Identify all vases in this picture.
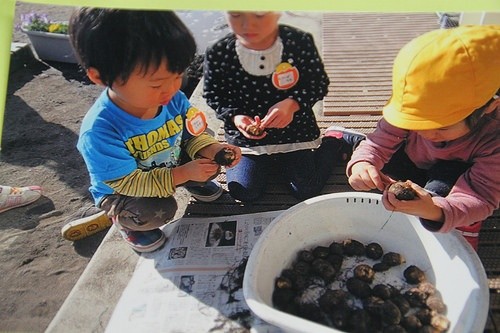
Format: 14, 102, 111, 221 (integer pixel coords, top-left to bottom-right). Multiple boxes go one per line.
21, 21, 85, 63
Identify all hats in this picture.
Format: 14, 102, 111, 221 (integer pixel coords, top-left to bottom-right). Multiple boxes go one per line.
382, 24, 500, 131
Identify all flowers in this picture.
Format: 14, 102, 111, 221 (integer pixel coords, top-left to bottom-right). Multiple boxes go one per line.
21, 14, 71, 35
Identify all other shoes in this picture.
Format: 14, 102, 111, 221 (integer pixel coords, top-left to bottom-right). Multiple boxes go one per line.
184, 179, 223, 203
0, 185, 42, 213
61, 205, 113, 241
456, 222, 480, 254
112, 216, 167, 253
322, 125, 368, 147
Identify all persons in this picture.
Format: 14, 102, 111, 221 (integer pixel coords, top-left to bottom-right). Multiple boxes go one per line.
201, 11, 368, 203
68, 7, 241, 253
0, 185, 42, 212
346, 23, 500, 252
